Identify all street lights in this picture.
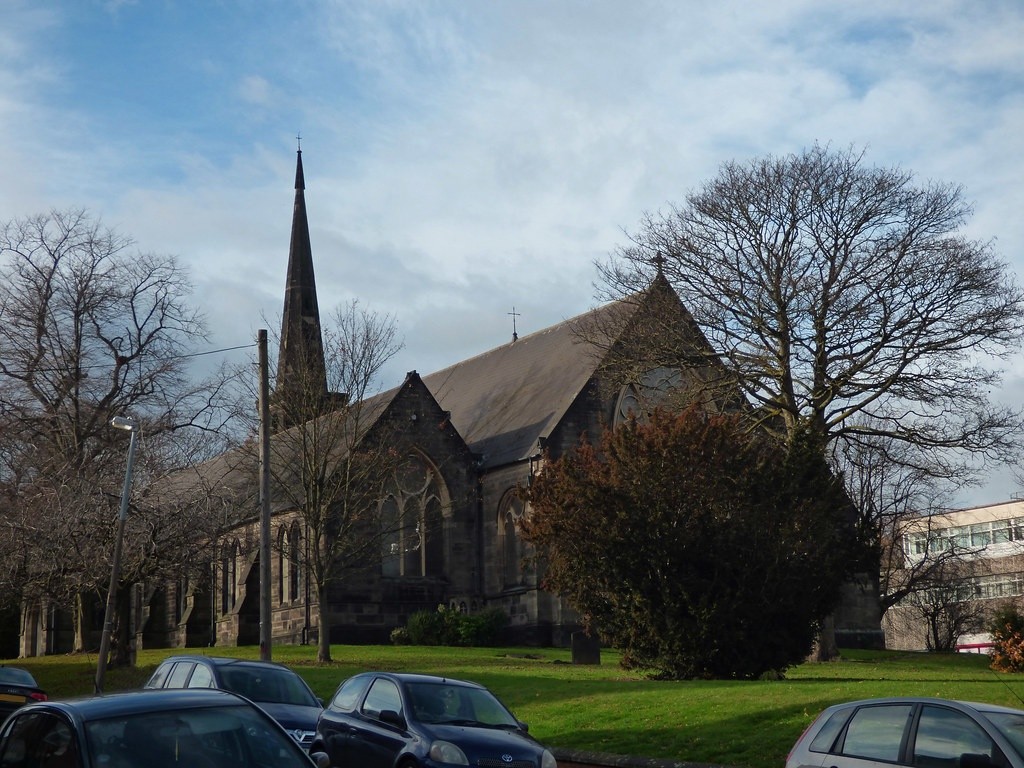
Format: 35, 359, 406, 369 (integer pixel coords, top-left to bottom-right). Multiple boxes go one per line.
90, 414, 140, 699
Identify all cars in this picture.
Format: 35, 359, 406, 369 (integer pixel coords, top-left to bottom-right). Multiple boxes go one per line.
0, 666, 48, 724
0, 685, 333, 768
307, 667, 558, 768
787, 693, 1024, 768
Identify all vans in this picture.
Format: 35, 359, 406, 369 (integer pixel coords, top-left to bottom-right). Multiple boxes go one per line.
143, 655, 328, 761
